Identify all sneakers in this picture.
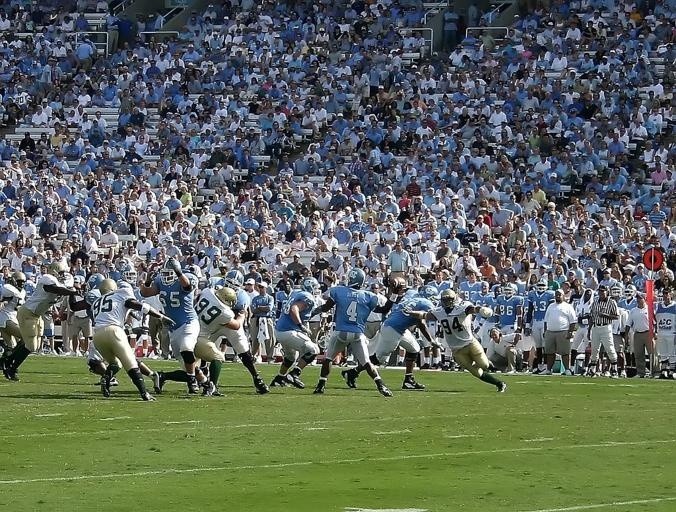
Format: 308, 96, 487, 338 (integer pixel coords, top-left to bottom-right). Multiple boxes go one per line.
35, 346, 414, 360
154, 371, 166, 394
186, 371, 228, 399
341, 369, 361, 388
421, 358, 676, 379
254, 366, 306, 395
373, 377, 395, 398
99, 375, 120, 398
141, 391, 158, 402
401, 373, 427, 391
313, 378, 326, 395
495, 378, 507, 393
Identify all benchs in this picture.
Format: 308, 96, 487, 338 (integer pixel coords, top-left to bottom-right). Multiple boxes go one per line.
1, 1, 676, 304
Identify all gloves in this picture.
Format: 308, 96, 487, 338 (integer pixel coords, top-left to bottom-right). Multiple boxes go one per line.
474, 303, 495, 319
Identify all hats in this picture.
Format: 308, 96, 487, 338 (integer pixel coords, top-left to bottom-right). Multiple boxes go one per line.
243, 275, 268, 288
1, 68, 616, 274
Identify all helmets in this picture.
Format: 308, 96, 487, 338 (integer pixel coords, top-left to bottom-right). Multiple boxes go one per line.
73, 272, 117, 294
300, 276, 322, 298
389, 276, 407, 297
215, 269, 245, 307
347, 267, 366, 288
502, 280, 638, 299
160, 258, 182, 286
420, 284, 440, 306
441, 288, 456, 310
